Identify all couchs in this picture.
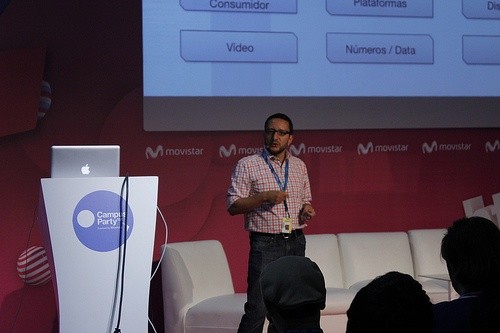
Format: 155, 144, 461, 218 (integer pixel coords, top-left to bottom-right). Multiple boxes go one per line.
303, 228, 460, 333
159, 240, 270, 333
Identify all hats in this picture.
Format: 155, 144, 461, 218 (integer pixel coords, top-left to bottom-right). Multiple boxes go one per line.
259, 255, 325, 309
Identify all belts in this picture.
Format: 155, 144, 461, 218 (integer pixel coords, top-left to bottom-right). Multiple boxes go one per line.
251, 229, 303, 239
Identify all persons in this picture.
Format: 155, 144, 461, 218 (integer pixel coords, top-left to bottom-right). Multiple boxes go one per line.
345, 216, 500, 333
259, 255, 326, 333
226, 113, 317, 333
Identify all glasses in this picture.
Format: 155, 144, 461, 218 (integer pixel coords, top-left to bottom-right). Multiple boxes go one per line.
265, 128, 291, 136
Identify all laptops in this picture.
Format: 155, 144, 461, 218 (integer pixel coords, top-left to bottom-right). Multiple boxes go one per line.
50, 146, 120, 178
